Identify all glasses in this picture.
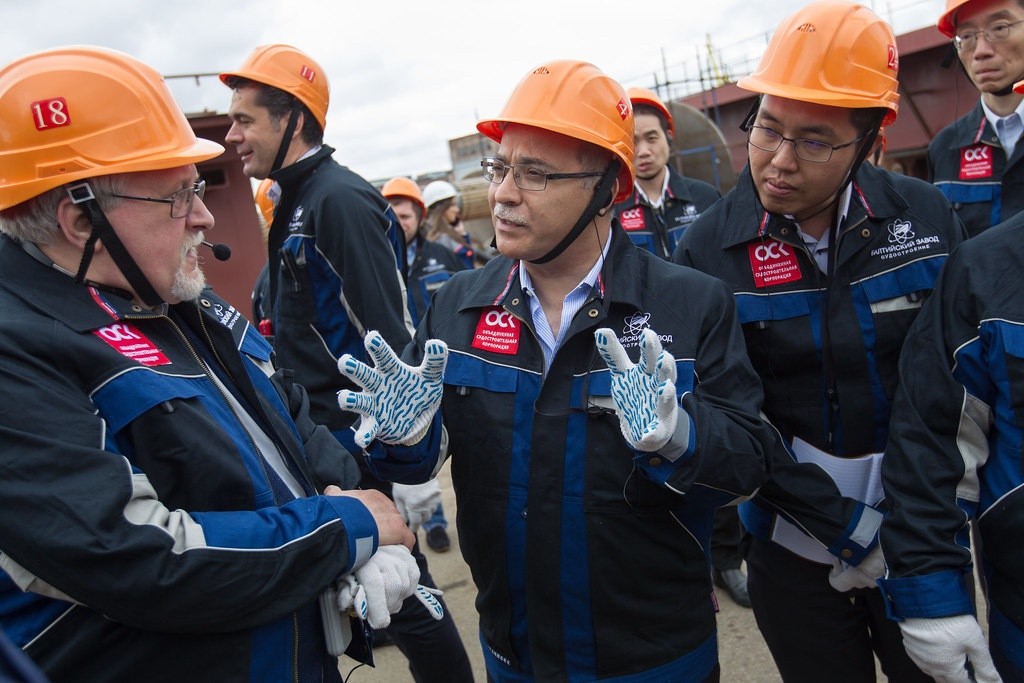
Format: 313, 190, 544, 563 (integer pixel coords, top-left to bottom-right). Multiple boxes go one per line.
744, 112, 866, 163
952, 20, 1024, 51
480, 157, 607, 191
104, 180, 206, 218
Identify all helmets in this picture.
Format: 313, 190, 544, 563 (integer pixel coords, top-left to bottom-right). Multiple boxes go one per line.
256, 179, 280, 225
627, 87, 674, 135
937, 0, 970, 38
219, 44, 328, 135
476, 59, 635, 204
381, 177, 425, 222
0, 44, 226, 208
422, 180, 456, 208
736, 1, 900, 126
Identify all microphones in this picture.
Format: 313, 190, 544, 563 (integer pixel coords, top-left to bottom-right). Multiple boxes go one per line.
200, 241, 231, 261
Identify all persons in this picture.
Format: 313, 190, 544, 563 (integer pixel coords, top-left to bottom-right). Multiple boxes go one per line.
880, 78, 1024, 683
380, 177, 472, 553
669, 0, 978, 683
338, 61, 775, 683
420, 181, 476, 268
0, 46, 416, 683
221, 45, 474, 683
254, 177, 279, 345
923, 0, 1024, 616
612, 87, 753, 609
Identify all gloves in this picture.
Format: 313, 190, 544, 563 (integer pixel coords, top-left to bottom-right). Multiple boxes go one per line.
394, 479, 442, 532
337, 330, 448, 447
596, 327, 678, 453
337, 542, 443, 628
898, 613, 1003, 683
828, 558, 879, 591
858, 541, 889, 583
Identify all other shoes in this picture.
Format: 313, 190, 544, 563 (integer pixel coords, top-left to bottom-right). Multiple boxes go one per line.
426, 527, 449, 551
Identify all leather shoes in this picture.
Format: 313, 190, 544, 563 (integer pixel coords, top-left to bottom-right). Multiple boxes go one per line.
713, 567, 752, 607
